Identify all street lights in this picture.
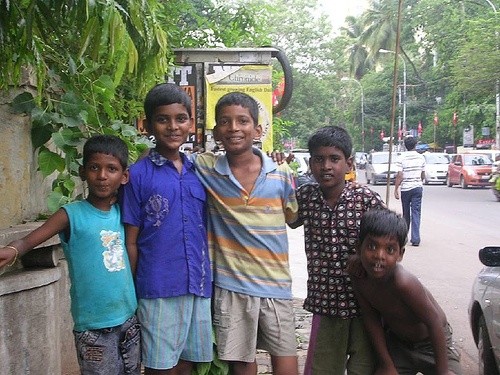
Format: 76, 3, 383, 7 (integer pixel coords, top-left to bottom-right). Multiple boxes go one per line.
378, 48, 407, 149
340, 77, 365, 154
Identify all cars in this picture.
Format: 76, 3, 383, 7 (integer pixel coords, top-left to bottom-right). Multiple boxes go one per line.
282, 148, 313, 175
341, 166, 358, 181
467, 246, 500, 375
352, 151, 402, 186
420, 152, 500, 202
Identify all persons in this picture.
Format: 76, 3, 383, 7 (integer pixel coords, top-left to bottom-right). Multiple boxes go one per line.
121, 83, 213, 375
268, 126, 388, 375
188, 92, 307, 375
288, 154, 301, 187
0, 134, 141, 375
350, 208, 465, 375
394, 138, 427, 246
